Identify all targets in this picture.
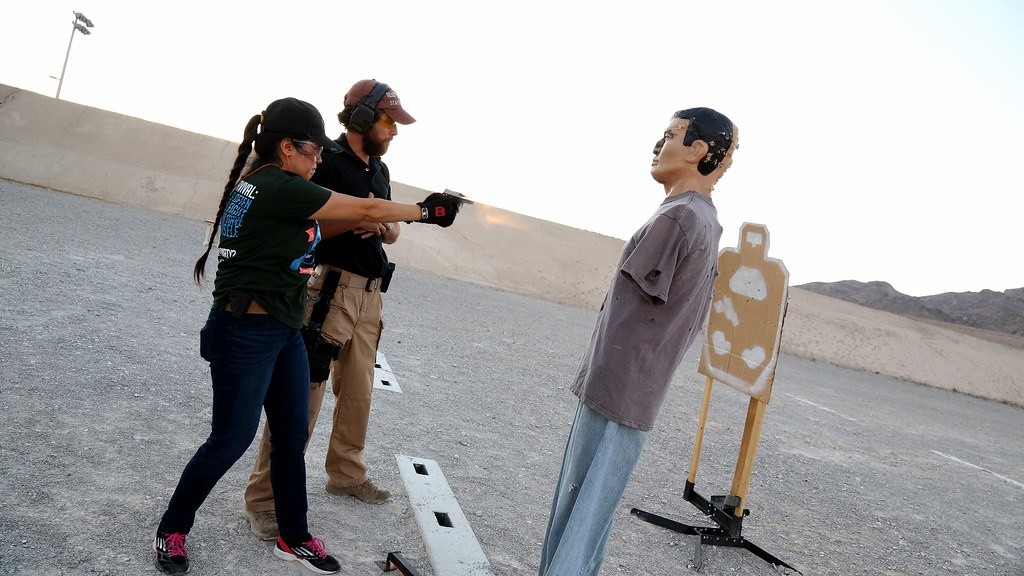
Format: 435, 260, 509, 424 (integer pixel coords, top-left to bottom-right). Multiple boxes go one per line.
630, 220, 806, 576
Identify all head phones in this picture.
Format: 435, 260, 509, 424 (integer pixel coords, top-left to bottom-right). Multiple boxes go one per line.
349, 83, 389, 133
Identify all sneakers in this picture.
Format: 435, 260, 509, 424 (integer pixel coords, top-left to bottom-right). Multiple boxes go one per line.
241, 503, 282, 542
152, 532, 192, 576
324, 477, 392, 505
272, 532, 342, 575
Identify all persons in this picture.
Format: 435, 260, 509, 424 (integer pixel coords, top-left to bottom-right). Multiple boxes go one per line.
243, 77, 417, 541
537, 107, 738, 576
153, 97, 474, 576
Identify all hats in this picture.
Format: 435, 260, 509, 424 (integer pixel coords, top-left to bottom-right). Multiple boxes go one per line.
258, 97, 346, 153
344, 81, 418, 128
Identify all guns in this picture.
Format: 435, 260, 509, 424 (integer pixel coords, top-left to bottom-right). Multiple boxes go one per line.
442, 188, 474, 217
